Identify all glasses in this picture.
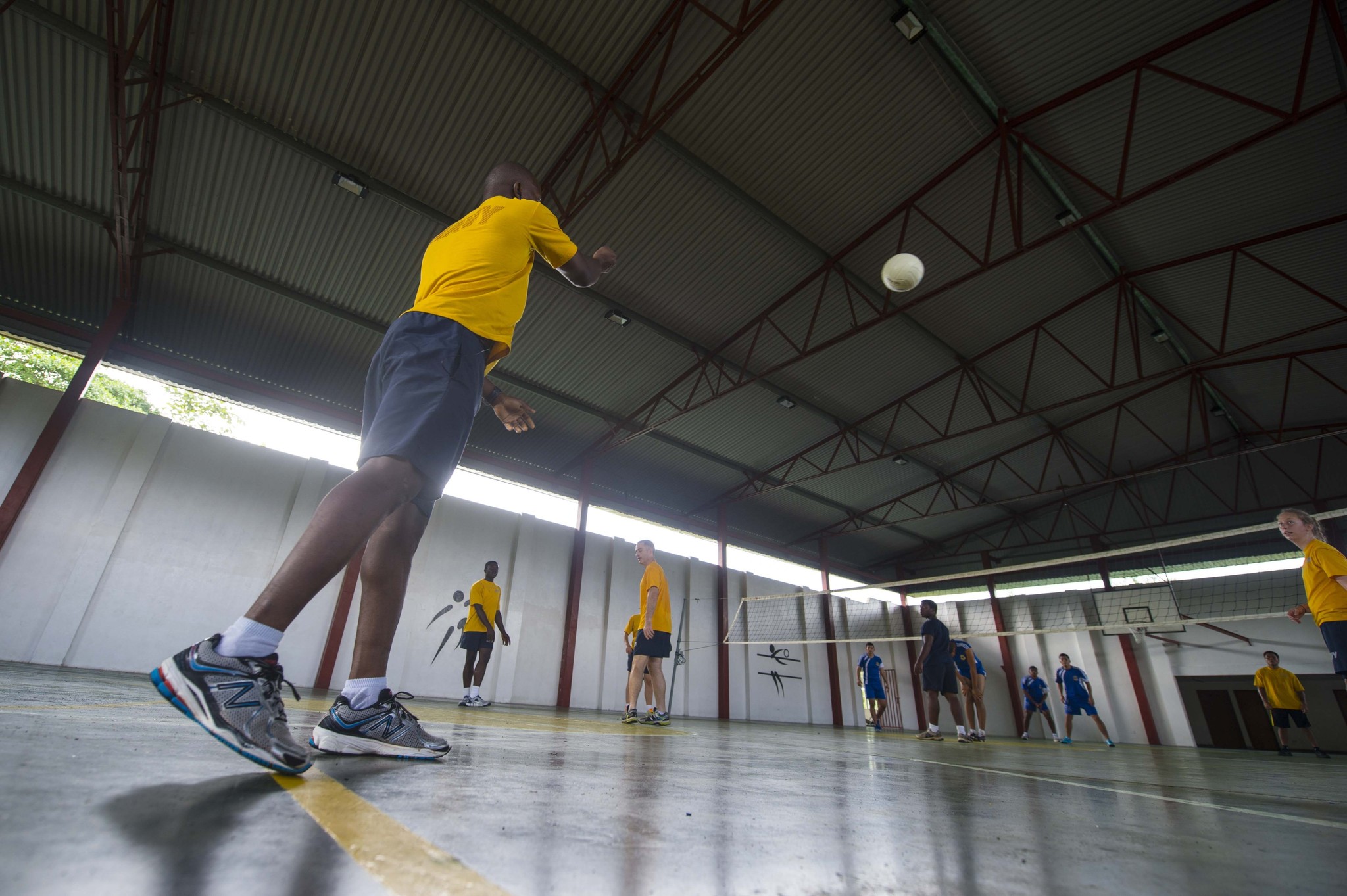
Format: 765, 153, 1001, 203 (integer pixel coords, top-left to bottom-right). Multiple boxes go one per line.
487, 567, 498, 570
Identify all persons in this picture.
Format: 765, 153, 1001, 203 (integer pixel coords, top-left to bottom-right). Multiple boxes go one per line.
913, 599, 974, 743
948, 639, 987, 742
620, 540, 672, 727
1276, 508, 1347, 686
1254, 651, 1332, 758
856, 642, 890, 731
1055, 653, 1115, 747
1021, 666, 1060, 742
150, 163, 614, 775
458, 561, 511, 707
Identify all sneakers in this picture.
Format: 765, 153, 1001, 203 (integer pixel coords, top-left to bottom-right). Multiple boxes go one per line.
638, 707, 670, 726
877, 719, 882, 730
458, 695, 470, 706
915, 728, 944, 741
957, 733, 974, 743
622, 704, 638, 724
875, 725, 881, 732
466, 695, 491, 707
148, 633, 316, 775
309, 688, 452, 759
645, 708, 654, 717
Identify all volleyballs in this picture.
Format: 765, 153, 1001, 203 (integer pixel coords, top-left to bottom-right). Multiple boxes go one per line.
882, 252, 925, 293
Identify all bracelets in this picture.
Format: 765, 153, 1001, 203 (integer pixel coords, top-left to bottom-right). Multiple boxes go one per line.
1298, 605, 1306, 613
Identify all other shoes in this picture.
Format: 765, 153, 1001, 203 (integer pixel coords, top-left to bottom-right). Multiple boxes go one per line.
1053, 737, 1060, 742
1022, 735, 1029, 740
1060, 736, 1073, 744
1106, 740, 1115, 747
978, 736, 986, 742
1278, 745, 1293, 756
968, 731, 979, 741
1313, 747, 1330, 758
